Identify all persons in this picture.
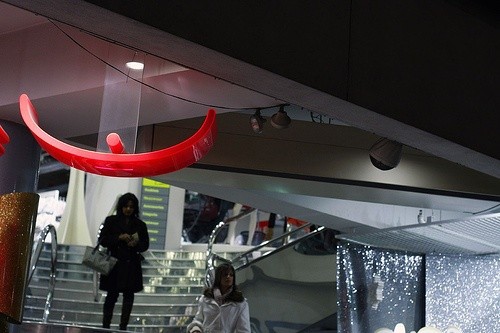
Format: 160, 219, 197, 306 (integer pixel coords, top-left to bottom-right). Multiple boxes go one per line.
96, 192, 149, 331
185, 263, 251, 333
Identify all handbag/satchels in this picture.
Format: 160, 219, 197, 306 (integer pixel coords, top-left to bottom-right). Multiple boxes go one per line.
81, 242, 119, 276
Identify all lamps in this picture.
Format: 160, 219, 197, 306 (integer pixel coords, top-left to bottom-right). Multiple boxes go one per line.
250, 109, 267, 136
270, 105, 290, 129
